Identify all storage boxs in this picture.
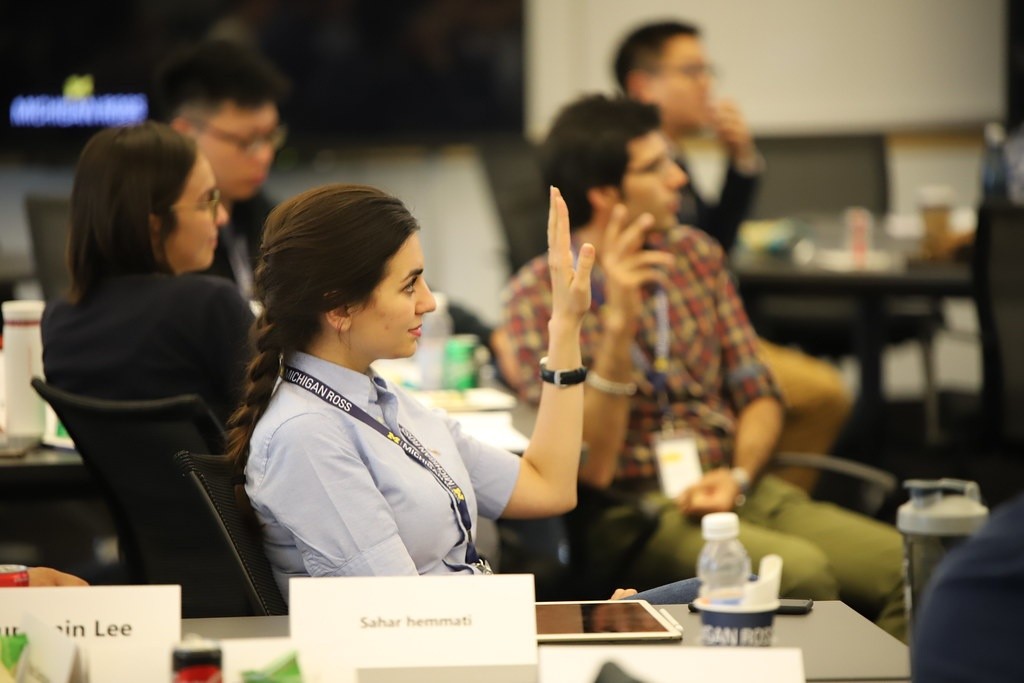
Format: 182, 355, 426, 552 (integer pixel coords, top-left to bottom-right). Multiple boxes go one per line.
286, 573, 539, 683
0, 583, 179, 683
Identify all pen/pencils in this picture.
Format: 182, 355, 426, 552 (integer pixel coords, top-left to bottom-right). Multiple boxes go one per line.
659, 608, 684, 632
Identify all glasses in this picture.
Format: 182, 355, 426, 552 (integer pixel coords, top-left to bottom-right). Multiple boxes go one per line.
655, 63, 717, 78
168, 189, 220, 224
629, 147, 675, 178
183, 116, 290, 154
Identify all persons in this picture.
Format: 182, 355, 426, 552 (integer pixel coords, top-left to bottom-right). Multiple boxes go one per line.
607, 13, 852, 496
39, 121, 284, 427
493, 93, 932, 643
905, 494, 1024, 683
134, 60, 290, 322
223, 184, 759, 616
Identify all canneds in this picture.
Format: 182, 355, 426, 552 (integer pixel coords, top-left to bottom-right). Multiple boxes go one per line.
0, 564, 30, 588
443, 334, 478, 390
169, 633, 223, 683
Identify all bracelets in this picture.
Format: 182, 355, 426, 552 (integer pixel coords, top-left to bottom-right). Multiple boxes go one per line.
589, 368, 640, 398
539, 358, 589, 389
729, 464, 752, 492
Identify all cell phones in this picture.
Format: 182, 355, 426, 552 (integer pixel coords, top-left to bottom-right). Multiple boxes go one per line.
688, 597, 814, 615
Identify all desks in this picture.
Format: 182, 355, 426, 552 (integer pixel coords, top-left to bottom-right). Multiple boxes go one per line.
723, 229, 978, 478
180, 596, 910, 683
0, 369, 587, 495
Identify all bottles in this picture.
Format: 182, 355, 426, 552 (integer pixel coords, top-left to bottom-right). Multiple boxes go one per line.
697, 511, 752, 609
0, 300, 46, 439
896, 477, 990, 638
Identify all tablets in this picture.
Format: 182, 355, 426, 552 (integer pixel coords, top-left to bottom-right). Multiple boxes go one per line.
535, 599, 682, 643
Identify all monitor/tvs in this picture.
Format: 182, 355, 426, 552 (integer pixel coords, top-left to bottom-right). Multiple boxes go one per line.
0, 0, 527, 151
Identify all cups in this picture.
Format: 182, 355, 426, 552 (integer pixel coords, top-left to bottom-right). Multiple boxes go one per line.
917, 183, 955, 258
691, 597, 782, 647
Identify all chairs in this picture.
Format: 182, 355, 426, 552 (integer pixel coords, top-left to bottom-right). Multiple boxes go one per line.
175, 441, 287, 618
28, 379, 244, 618
